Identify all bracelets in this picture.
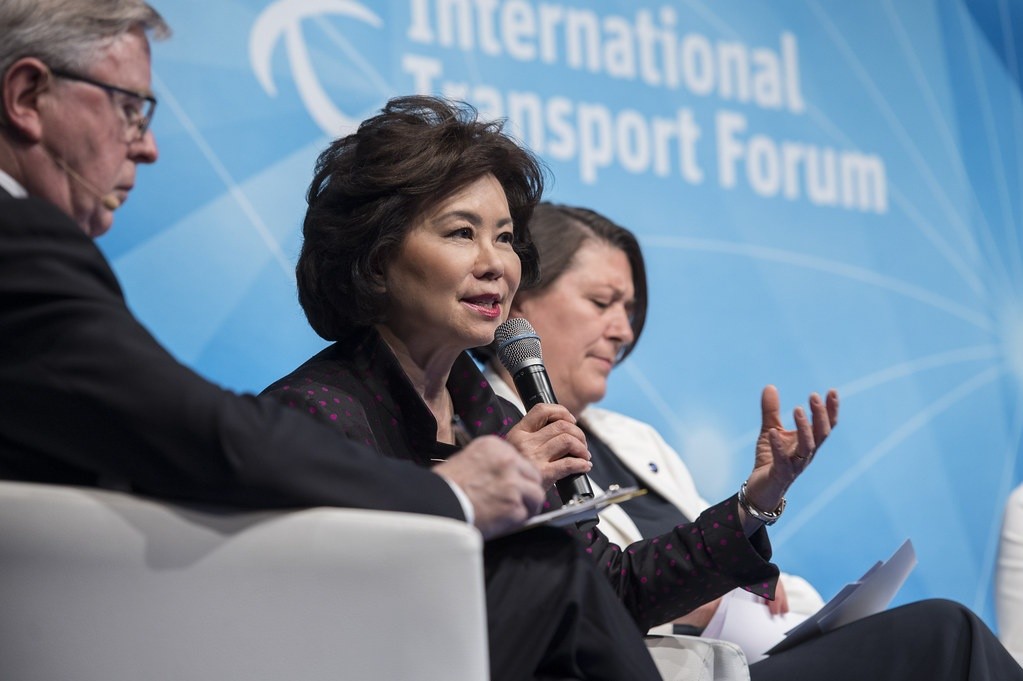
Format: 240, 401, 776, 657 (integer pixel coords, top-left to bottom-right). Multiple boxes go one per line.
740, 481, 787, 526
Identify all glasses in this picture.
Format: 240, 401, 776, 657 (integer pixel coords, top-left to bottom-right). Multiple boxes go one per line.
52, 67, 157, 139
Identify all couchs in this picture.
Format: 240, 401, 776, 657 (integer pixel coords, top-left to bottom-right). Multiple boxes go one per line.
0, 481, 825, 681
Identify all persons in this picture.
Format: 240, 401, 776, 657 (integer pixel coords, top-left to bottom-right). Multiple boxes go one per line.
0, 0, 662, 681
259, 96, 840, 637
470, 202, 1023, 681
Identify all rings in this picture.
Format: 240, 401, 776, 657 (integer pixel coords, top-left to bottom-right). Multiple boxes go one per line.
793, 453, 812, 464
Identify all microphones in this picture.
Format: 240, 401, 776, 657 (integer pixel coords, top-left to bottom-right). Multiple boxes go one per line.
495, 317, 600, 533
38, 142, 122, 213
